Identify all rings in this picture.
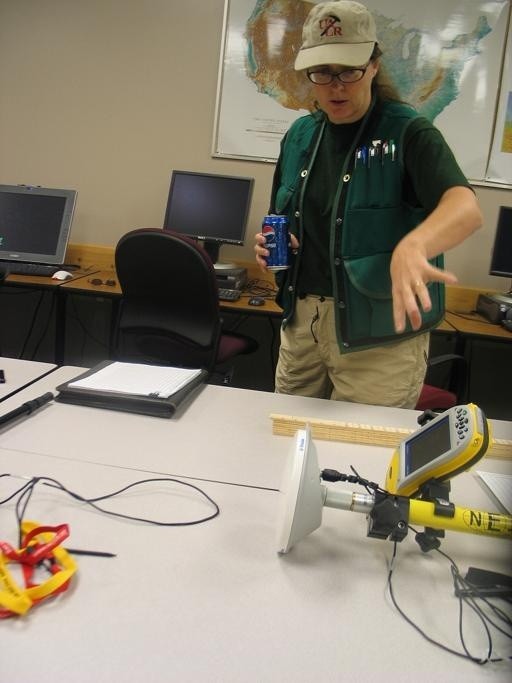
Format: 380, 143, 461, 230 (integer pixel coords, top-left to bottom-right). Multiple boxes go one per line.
413, 280, 424, 289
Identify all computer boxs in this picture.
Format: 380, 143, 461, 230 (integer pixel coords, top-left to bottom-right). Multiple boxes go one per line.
215, 269, 248, 291
476, 294, 512, 326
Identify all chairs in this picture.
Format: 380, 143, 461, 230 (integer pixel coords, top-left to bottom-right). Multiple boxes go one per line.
413, 382, 459, 412
114, 226, 260, 381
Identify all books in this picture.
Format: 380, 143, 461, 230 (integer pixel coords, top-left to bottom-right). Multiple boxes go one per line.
53, 359, 208, 419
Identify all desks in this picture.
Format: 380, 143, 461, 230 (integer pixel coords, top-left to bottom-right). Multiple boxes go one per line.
0, 244, 110, 294
444, 282, 512, 404
59, 242, 459, 402
0, 356, 512, 683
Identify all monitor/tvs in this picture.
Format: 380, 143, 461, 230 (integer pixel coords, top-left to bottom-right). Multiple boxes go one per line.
162, 170, 256, 269
476, 205, 512, 303
0, 186, 79, 265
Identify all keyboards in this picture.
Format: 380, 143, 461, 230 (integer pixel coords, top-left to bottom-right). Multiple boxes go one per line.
218, 288, 242, 302
0, 259, 59, 275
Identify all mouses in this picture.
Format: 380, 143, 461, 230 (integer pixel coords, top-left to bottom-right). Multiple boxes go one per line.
51, 270, 74, 280
249, 297, 265, 306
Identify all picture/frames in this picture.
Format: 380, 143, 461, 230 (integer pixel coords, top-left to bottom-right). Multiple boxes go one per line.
210, 0, 512, 190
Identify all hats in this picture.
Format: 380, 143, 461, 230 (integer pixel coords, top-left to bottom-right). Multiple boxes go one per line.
293, 1, 379, 73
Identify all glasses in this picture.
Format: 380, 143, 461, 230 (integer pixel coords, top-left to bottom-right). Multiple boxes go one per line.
305, 53, 377, 86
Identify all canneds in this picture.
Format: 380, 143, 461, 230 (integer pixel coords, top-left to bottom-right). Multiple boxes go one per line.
262, 216, 291, 272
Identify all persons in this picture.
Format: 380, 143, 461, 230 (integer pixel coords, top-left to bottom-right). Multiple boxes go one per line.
254, 1, 485, 410
